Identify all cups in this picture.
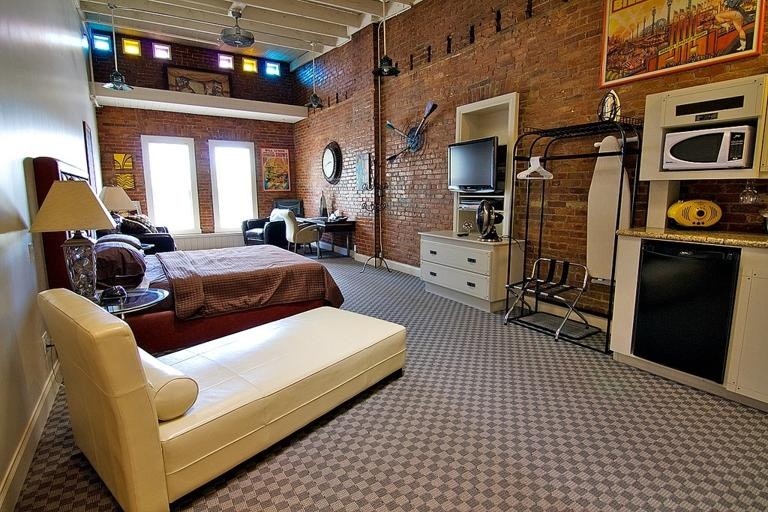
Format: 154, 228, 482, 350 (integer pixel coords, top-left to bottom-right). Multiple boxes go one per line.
336, 210, 343, 216
464, 217, 473, 234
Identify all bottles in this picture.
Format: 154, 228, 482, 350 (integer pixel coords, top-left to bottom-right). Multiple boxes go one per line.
329, 191, 336, 212
318, 190, 327, 216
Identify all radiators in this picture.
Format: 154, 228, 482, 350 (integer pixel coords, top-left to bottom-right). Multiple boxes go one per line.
173, 232, 244, 250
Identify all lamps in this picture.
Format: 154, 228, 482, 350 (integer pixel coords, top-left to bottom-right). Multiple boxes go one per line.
27, 180, 117, 304
102, 4, 134, 92
372, 0, 400, 76
99, 187, 137, 232
221, 11, 254, 47
304, 42, 325, 109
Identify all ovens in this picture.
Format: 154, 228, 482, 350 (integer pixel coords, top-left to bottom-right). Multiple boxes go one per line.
633, 239, 742, 386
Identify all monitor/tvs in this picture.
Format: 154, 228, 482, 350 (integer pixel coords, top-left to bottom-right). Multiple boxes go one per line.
447, 136, 499, 194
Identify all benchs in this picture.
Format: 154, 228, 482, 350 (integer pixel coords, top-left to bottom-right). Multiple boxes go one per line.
37, 288, 407, 512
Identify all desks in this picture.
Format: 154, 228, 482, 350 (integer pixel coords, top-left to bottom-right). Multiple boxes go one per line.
303, 218, 356, 258
94, 289, 169, 318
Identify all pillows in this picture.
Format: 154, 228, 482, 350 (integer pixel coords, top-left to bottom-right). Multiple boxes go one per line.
96, 234, 145, 257
97, 242, 147, 288
125, 215, 159, 233
137, 346, 199, 422
269, 208, 288, 222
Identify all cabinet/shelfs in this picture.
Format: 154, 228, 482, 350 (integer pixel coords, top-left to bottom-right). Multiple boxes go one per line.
417, 231, 525, 314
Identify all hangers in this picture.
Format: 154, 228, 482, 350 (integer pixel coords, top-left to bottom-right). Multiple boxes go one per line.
516, 157, 553, 180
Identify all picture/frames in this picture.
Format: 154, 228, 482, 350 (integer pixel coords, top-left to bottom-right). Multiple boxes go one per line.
599, 0, 766, 89
83, 121, 97, 191
163, 64, 233, 98
261, 148, 292, 192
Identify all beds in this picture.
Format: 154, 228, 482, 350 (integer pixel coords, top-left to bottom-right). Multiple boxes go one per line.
33, 157, 345, 354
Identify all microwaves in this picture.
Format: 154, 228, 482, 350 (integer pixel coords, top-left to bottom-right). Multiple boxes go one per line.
662, 125, 753, 171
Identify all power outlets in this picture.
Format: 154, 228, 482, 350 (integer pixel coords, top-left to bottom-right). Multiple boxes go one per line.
41, 331, 52, 359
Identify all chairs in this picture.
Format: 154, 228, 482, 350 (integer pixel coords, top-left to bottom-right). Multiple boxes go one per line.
241, 209, 287, 249
122, 215, 176, 254
503, 258, 601, 341
280, 211, 322, 260
